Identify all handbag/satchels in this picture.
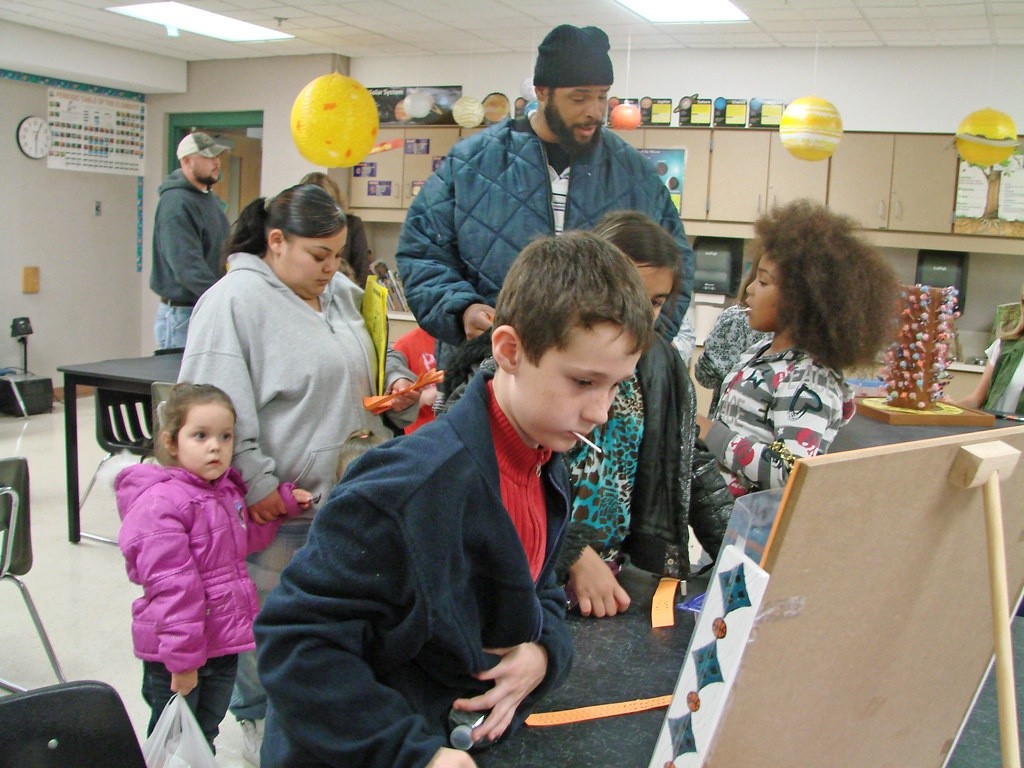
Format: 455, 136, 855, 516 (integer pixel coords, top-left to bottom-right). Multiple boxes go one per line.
145, 695, 219, 768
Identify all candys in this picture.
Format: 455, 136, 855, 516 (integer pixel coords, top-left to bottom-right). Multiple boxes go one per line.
878, 285, 961, 408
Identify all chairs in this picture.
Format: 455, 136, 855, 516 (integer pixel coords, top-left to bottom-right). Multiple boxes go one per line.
0, 457, 68, 694
79, 388, 156, 546
0, 680, 146, 768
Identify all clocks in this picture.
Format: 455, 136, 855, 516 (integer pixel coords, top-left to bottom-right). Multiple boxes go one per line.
17, 116, 53, 159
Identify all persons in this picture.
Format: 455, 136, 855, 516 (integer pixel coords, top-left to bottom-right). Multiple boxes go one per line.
148, 132, 234, 356
940, 279, 1024, 415
445, 200, 750, 618
250, 229, 655, 768
696, 194, 903, 571
396, 24, 696, 418
176, 182, 430, 768
385, 324, 439, 436
112, 379, 315, 757
297, 172, 373, 291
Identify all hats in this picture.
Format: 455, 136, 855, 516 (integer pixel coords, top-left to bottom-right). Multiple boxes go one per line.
176, 132, 231, 158
533, 24, 614, 86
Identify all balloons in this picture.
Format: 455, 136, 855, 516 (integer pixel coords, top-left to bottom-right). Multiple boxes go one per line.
403, 92, 433, 117
609, 103, 643, 130
779, 96, 843, 162
955, 109, 1017, 167
290, 71, 380, 169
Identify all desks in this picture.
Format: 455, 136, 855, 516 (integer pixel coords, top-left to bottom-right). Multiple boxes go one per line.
56, 353, 185, 545
466, 565, 1024, 768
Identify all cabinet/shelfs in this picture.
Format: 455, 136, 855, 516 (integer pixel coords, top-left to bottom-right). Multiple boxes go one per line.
347, 122, 1024, 254
